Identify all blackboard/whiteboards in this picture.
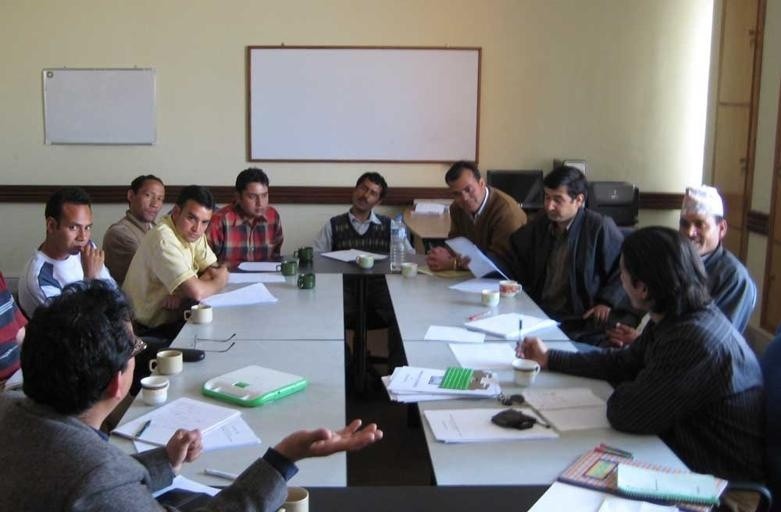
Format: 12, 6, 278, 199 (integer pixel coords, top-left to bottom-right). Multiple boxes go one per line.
42, 67, 156, 145
247, 47, 481, 164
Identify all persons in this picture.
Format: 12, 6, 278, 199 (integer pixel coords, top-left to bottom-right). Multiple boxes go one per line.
598, 181, 758, 352
422, 159, 529, 273
119, 184, 229, 345
204, 166, 285, 268
512, 222, 768, 482
504, 164, 629, 332
16, 187, 166, 380
301, 169, 413, 256
101, 172, 165, 283
0, 269, 27, 392
0, 275, 385, 512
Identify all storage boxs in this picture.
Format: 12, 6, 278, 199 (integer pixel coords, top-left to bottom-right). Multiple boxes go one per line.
400, 206, 453, 255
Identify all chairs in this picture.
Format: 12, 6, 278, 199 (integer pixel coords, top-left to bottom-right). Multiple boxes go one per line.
718, 328, 781, 512
735, 278, 757, 336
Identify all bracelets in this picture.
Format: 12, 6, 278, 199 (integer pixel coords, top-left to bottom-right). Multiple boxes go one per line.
450, 254, 466, 270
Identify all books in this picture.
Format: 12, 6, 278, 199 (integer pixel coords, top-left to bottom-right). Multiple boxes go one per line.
613, 464, 720, 507
465, 311, 560, 340
556, 450, 729, 511
111, 396, 242, 447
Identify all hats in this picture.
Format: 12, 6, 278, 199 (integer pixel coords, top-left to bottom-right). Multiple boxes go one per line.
679, 184, 724, 217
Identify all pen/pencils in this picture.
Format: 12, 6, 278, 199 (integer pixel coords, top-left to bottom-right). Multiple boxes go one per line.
136, 419, 151, 437
519, 316, 523, 347
428, 241, 434, 250
601, 442, 633, 455
538, 421, 549, 428
203, 468, 237, 481
594, 446, 632, 458
469, 310, 491, 321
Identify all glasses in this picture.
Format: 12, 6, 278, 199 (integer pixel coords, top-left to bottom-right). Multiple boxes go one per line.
101, 333, 147, 392
193, 334, 236, 353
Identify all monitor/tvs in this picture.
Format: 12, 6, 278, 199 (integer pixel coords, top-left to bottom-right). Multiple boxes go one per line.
486, 168, 544, 212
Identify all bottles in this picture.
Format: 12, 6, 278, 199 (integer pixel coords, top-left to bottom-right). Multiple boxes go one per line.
389, 215, 405, 272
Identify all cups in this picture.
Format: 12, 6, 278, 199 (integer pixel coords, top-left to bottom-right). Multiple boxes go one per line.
276, 259, 298, 276
499, 280, 522, 297
149, 350, 183, 375
294, 247, 314, 263
140, 376, 169, 405
400, 262, 417, 278
183, 304, 213, 324
511, 359, 541, 386
356, 255, 375, 269
276, 486, 309, 512
298, 273, 316, 289
481, 289, 500, 307
